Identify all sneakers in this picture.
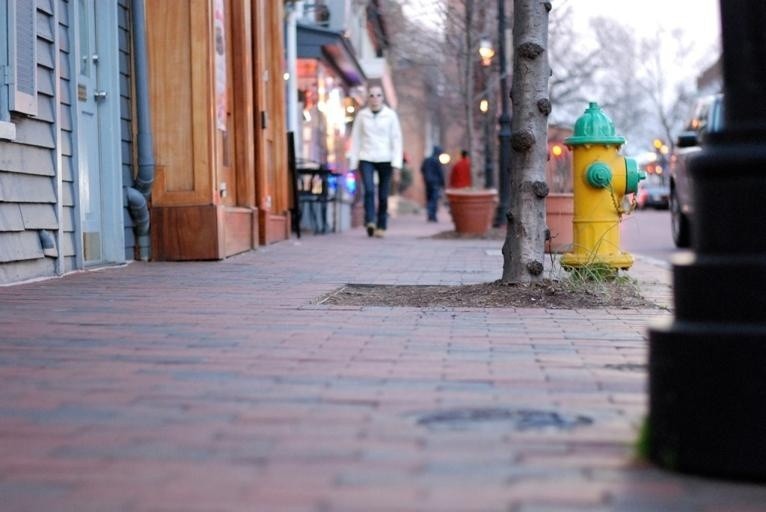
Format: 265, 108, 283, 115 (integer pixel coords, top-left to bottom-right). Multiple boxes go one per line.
366, 223, 384, 237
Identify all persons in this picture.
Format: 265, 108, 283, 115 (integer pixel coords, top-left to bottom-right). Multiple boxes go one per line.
421, 142, 447, 222
449, 148, 473, 189
349, 83, 404, 239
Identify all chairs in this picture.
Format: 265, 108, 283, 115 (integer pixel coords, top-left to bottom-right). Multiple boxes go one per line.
295, 168, 343, 233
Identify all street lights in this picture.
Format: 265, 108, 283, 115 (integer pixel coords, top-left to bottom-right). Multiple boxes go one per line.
479, 47, 495, 189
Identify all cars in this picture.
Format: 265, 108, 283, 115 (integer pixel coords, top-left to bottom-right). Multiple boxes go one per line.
638, 92, 724, 250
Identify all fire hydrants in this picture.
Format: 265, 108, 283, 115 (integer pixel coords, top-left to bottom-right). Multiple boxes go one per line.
559, 101, 646, 284
